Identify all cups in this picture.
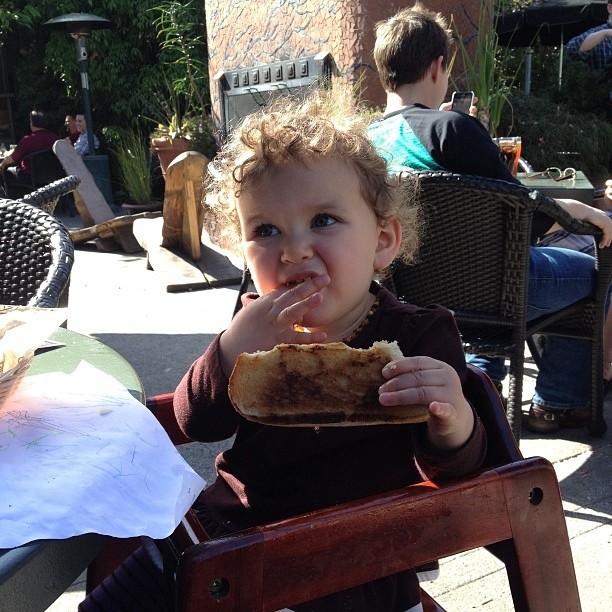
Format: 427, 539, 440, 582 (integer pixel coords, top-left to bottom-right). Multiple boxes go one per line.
491, 136, 517, 175
500, 136, 522, 173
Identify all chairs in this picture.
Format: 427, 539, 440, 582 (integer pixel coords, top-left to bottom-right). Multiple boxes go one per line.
0, 199, 74, 308
181, 456, 578, 610
20, 173, 81, 214
86, 391, 213, 599
381, 170, 611, 450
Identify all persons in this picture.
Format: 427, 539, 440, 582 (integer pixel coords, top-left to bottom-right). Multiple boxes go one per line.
77, 89, 503, 612
0, 111, 59, 191
64, 113, 81, 147
534, 178, 612, 399
566, 0, 612, 71
363, 2, 612, 433
74, 113, 100, 157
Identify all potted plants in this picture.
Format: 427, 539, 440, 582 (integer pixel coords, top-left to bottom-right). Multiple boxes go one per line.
105, 126, 160, 216
138, 71, 203, 184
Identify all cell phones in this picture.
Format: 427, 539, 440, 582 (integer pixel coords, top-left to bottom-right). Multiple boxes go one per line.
451, 90, 474, 117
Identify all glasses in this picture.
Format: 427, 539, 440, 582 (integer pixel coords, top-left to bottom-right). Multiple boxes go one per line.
526, 166, 577, 181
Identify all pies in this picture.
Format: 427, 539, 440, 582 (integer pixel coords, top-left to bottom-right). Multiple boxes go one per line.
226, 339, 433, 428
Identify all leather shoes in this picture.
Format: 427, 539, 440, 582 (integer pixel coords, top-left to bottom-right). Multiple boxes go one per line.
492, 380, 507, 413
526, 404, 594, 434
